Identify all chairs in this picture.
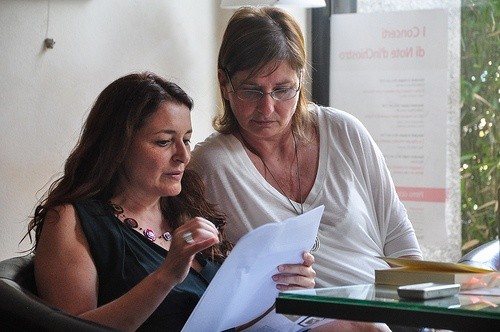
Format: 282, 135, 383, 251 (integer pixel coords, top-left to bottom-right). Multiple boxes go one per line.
0, 254, 123, 332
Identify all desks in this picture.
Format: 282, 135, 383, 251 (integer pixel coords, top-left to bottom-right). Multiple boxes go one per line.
275, 283, 500, 332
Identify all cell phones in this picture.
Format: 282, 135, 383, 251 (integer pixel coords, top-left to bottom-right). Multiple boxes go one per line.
396, 283, 460, 300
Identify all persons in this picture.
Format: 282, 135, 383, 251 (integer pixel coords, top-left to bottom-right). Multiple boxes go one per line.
19, 72, 393, 332
374, 256, 500, 290
184, 6, 455, 332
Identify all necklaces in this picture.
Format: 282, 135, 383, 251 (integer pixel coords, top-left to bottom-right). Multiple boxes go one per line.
112, 203, 172, 243
243, 128, 321, 253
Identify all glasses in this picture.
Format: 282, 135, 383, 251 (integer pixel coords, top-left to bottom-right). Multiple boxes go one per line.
223, 69, 301, 102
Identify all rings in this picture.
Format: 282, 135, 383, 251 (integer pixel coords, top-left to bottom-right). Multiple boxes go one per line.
182, 233, 194, 244
